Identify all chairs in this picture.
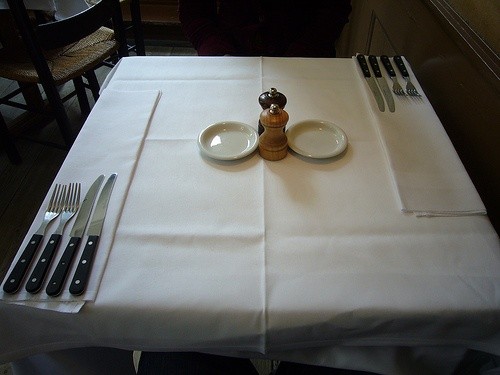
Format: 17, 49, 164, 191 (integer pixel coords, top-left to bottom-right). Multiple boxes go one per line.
1, 0, 130, 164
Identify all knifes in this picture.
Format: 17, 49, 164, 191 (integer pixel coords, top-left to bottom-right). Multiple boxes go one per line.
357, 54, 385, 112
368, 55, 395, 112
46, 175, 106, 297
69, 173, 118, 297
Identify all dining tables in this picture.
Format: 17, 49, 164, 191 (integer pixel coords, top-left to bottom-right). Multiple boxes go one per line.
0, 54, 500, 361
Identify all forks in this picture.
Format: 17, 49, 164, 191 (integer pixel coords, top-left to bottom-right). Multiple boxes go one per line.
3, 183, 67, 293
25, 182, 81, 294
380, 55, 408, 96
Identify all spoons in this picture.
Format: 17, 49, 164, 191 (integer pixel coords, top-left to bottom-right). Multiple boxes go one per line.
393, 56, 422, 96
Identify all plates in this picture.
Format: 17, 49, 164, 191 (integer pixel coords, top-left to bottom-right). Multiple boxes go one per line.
285, 119, 347, 159
199, 121, 260, 161
